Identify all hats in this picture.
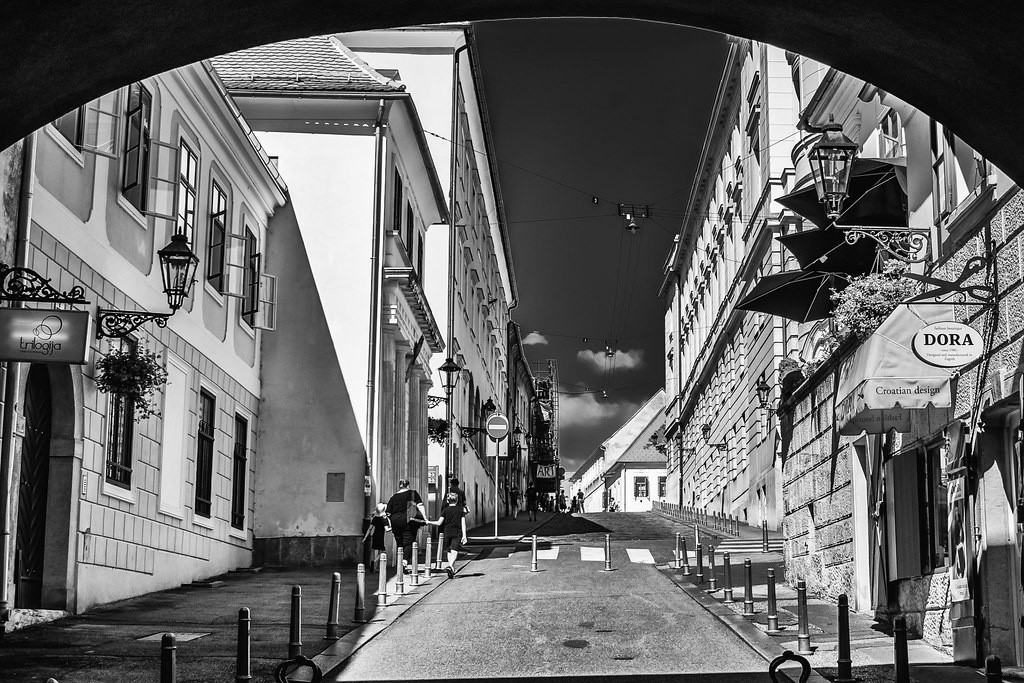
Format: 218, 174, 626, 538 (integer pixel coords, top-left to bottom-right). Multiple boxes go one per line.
373, 503, 387, 517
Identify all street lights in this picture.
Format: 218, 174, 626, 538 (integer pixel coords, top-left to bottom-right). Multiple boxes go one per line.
437, 358, 462, 497
524, 433, 534, 487
513, 427, 522, 487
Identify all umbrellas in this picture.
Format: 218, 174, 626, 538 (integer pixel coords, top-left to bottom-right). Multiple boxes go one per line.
736, 157, 908, 322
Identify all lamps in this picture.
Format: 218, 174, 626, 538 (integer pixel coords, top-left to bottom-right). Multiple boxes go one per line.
428, 355, 462, 409
651, 432, 669, 457
756, 381, 775, 411
96, 226, 199, 340
807, 112, 933, 268
522, 432, 534, 450
675, 433, 696, 455
701, 424, 728, 453
509, 426, 523, 454
461, 395, 496, 437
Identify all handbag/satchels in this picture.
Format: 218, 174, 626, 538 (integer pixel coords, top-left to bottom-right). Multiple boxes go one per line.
406, 490, 426, 526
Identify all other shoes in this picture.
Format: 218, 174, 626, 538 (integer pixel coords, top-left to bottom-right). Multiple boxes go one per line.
370, 560, 377, 574
446, 566, 453, 579
402, 562, 409, 574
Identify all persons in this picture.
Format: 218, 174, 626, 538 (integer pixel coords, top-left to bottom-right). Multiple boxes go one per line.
609, 497, 616, 512
362, 479, 470, 579
511, 482, 585, 522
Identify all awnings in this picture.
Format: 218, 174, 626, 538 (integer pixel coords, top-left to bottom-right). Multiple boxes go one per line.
834, 286, 956, 434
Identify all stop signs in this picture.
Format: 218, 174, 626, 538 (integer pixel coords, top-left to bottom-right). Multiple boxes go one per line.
488, 415, 509, 440
